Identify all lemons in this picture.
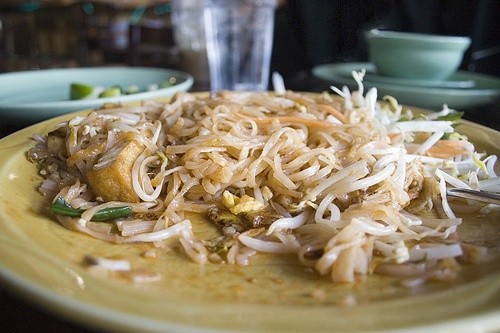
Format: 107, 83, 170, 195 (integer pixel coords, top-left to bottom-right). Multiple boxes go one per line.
70, 83, 121, 99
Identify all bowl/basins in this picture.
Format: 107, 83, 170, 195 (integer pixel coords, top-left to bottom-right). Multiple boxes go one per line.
363, 29, 471, 81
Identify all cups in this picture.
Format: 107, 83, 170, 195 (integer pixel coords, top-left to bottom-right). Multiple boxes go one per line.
171, 0, 272, 90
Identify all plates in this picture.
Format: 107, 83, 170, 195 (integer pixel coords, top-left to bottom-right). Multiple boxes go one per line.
0, 67, 196, 119
0, 91, 500, 333
311, 62, 500, 108
